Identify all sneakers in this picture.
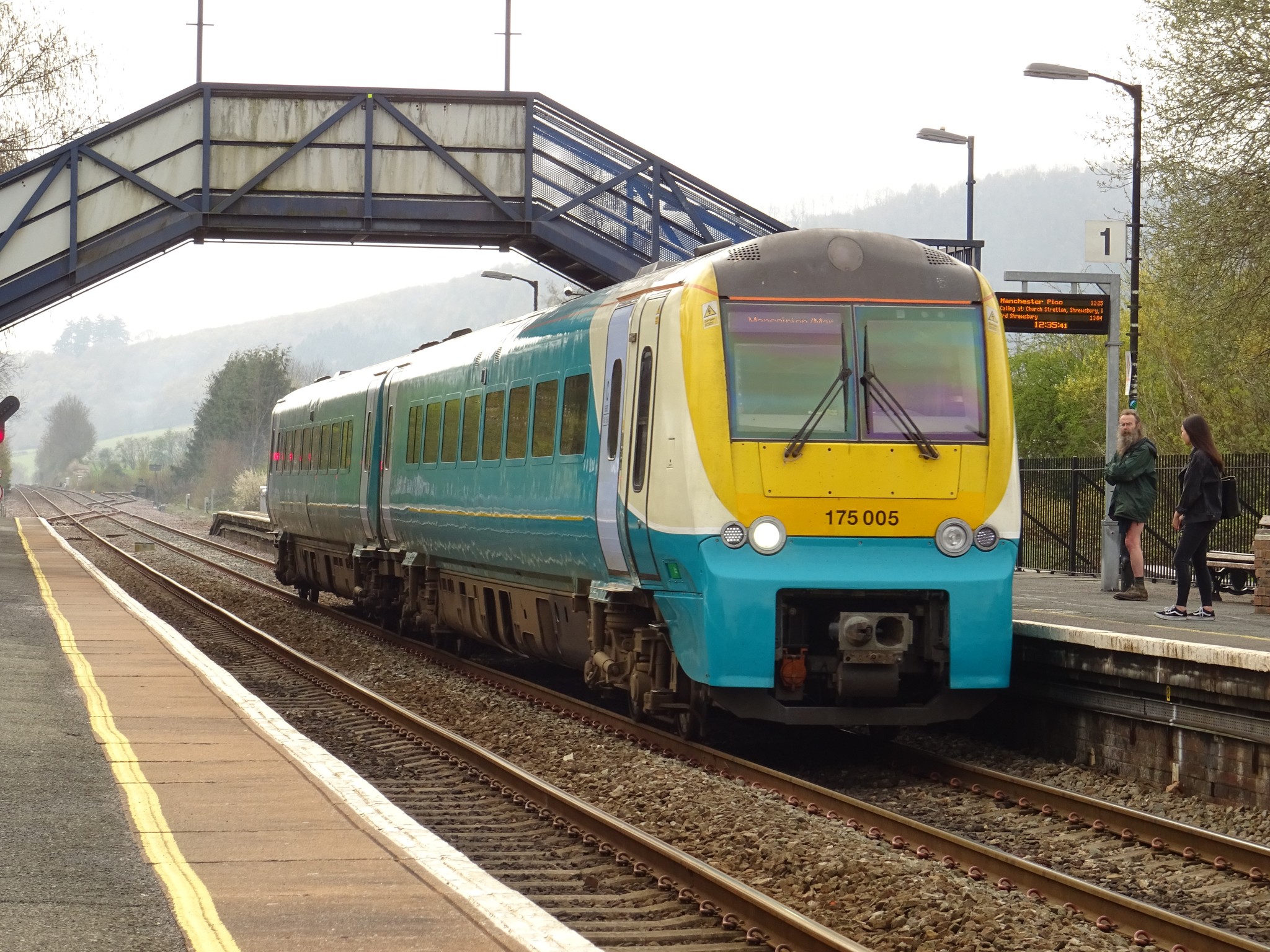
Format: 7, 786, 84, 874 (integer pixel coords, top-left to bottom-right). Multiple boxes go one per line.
1113, 587, 1148, 602
1154, 605, 1187, 621
1187, 606, 1216, 621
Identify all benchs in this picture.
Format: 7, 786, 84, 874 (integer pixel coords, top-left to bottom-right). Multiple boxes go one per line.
1207, 550, 1255, 601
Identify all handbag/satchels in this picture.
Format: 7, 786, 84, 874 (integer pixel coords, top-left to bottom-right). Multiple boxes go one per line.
1220, 472, 1241, 519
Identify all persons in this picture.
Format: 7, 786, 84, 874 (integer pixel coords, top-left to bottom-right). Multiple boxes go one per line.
1104, 409, 1159, 601
1153, 414, 1224, 621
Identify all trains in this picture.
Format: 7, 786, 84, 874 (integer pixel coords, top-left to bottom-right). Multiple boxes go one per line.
266, 230, 1024, 737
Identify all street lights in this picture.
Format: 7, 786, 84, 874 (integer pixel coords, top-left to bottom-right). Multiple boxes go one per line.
479, 270, 540, 312
917, 127, 976, 242
1023, 61, 1144, 421
